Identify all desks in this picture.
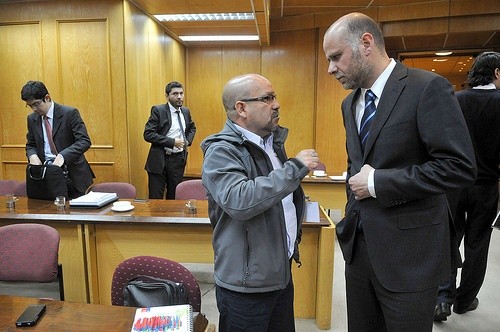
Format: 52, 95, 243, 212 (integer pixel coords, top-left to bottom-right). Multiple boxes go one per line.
0, 194, 336, 332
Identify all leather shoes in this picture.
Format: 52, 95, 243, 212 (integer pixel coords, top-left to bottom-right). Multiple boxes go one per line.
453, 298, 479, 314
434, 303, 452, 321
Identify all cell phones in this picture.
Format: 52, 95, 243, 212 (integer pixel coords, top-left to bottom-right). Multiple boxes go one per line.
15, 305, 46, 327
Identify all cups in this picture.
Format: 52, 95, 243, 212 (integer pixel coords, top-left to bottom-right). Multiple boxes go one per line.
111, 200, 135, 211
188, 197, 197, 214
54, 196, 66, 207
4, 194, 16, 213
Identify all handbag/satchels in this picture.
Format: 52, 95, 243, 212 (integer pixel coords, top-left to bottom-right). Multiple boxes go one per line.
26, 160, 70, 201
122, 274, 187, 308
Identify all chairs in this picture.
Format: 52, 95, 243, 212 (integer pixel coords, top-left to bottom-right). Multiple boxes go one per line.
175, 179, 209, 200
0, 223, 65, 302
0, 179, 27, 197
91, 182, 137, 198
110, 256, 202, 313
310, 161, 326, 173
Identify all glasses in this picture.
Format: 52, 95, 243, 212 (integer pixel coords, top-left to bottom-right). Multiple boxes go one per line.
25, 96, 44, 107
233, 95, 276, 110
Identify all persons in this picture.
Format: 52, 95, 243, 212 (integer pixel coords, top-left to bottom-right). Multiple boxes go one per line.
322, 12, 500, 331
143, 82, 196, 199
21, 80, 96, 200
199, 73, 319, 332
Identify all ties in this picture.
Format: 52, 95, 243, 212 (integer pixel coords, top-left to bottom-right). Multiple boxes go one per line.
175, 110, 187, 141
43, 115, 59, 156
360, 89, 377, 154
